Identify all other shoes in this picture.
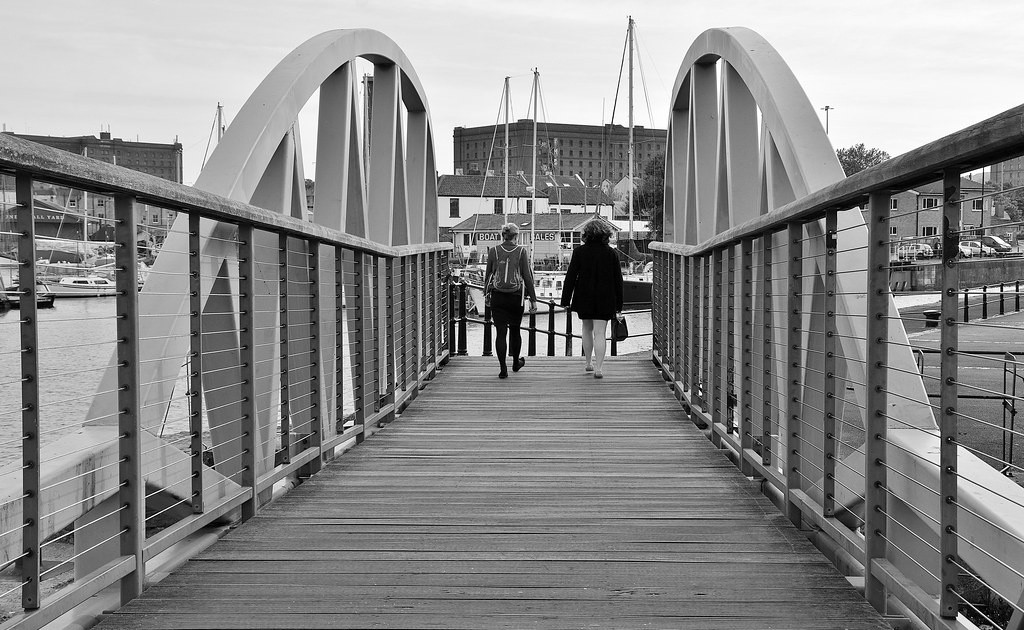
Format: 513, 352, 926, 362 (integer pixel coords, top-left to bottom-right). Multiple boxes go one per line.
586, 365, 594, 371
513, 357, 525, 372
499, 373, 508, 379
594, 372, 603, 378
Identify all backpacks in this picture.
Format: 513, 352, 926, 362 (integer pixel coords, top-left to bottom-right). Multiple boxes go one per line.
492, 244, 524, 293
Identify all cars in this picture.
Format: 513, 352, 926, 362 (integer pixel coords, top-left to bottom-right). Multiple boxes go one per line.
934, 242, 942, 258
976, 235, 1013, 252
897, 244, 934, 259
959, 241, 996, 258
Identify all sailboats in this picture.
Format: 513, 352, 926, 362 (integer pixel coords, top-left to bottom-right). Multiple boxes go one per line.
590, 16, 663, 313
450, 75, 510, 307
1, 144, 147, 310
464, 67, 573, 317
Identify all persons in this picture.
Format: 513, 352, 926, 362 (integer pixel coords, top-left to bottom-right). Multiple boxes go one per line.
484, 223, 537, 378
561, 219, 624, 377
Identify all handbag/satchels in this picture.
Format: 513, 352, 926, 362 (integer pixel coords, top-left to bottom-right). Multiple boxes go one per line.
612, 311, 628, 341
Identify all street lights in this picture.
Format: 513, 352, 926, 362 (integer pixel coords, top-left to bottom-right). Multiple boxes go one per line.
819, 105, 835, 136
